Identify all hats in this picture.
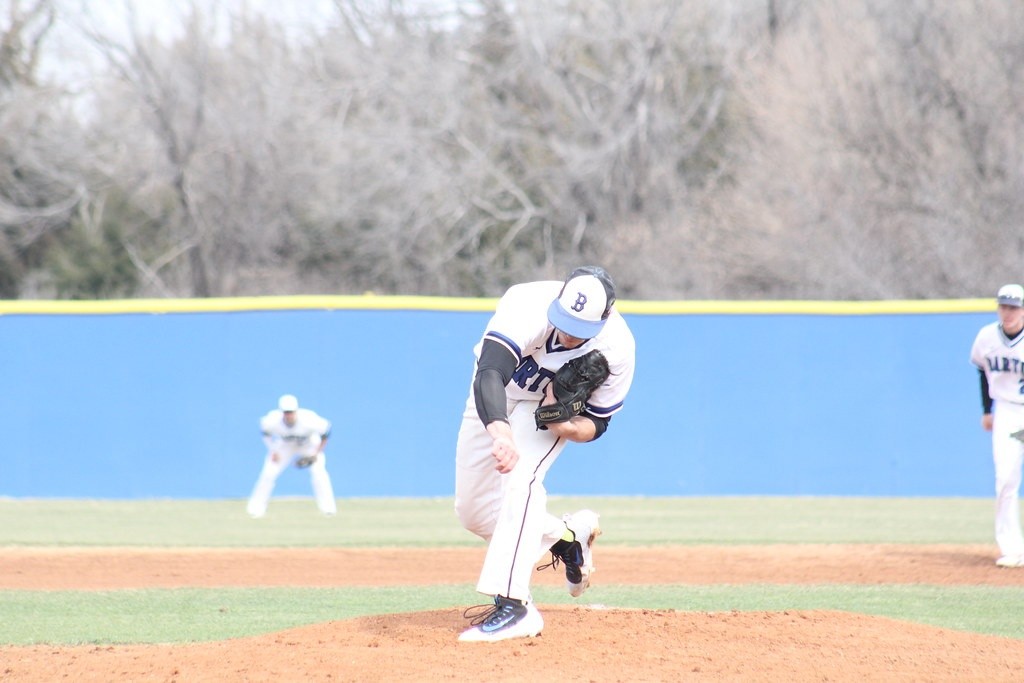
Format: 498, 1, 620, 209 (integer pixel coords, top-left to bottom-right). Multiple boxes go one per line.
547, 266, 616, 339
997, 284, 1024, 307
281, 395, 297, 411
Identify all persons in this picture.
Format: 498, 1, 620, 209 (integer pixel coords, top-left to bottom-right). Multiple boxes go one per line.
246, 395, 337, 519
969, 283, 1024, 568
455, 264, 637, 641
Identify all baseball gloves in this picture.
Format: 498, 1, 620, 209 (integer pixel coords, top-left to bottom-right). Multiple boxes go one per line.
535, 349, 610, 430
297, 456, 317, 468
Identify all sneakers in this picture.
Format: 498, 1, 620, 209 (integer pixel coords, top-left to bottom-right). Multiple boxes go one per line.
996, 556, 1024, 567
536, 509, 602, 597
458, 594, 544, 643
